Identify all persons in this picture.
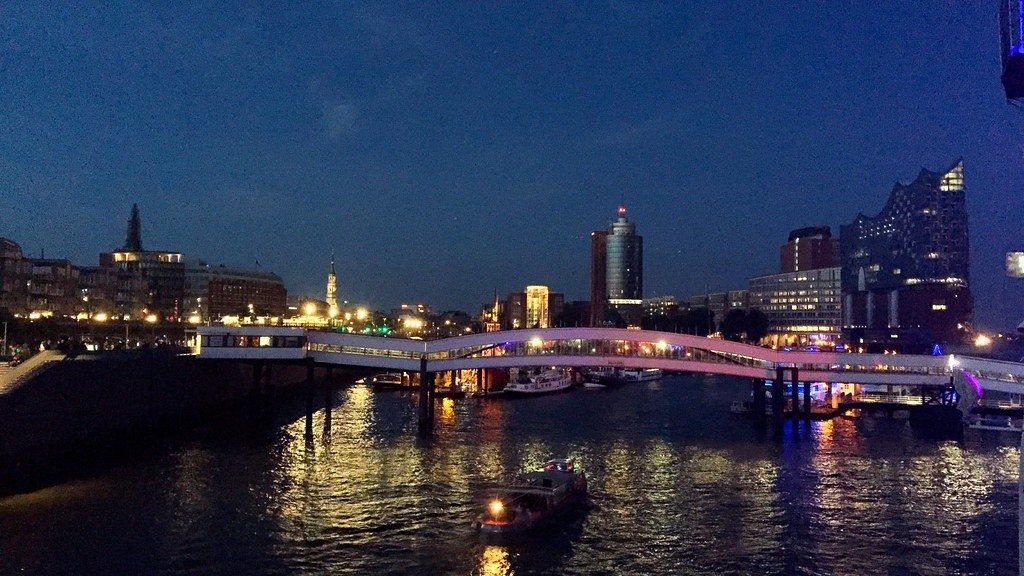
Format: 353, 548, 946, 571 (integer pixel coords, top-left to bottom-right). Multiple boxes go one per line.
155, 337, 180, 348
791, 338, 797, 347
40, 339, 65, 352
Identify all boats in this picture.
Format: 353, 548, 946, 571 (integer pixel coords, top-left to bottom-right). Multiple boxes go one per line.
504, 366, 573, 399
732, 381, 831, 416
582, 366, 662, 392
470, 456, 590, 535
373, 374, 402, 386
